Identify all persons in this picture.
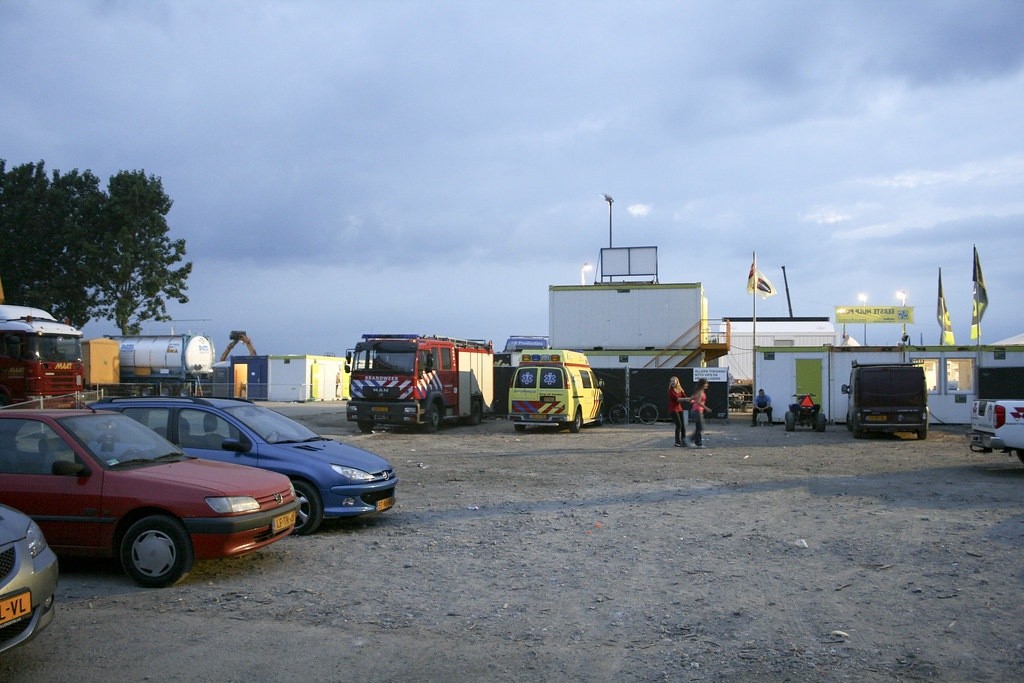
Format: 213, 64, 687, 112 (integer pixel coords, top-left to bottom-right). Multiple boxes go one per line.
669, 376, 694, 447
750, 389, 775, 427
689, 378, 712, 449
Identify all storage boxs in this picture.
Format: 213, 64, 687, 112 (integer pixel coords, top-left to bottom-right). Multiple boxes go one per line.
212, 366, 228, 398
268, 355, 353, 402
229, 354, 268, 400
80, 339, 120, 385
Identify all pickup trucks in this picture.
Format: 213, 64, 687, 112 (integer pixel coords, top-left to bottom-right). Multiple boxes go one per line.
966, 399, 1024, 465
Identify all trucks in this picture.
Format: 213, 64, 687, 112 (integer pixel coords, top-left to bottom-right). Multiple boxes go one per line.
0, 304, 84, 412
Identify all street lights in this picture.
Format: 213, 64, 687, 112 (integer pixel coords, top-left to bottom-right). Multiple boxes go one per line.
858, 293, 867, 346
599, 192, 614, 282
581, 262, 592, 285
897, 291, 906, 343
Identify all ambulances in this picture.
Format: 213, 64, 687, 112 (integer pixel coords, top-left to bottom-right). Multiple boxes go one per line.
509, 349, 605, 432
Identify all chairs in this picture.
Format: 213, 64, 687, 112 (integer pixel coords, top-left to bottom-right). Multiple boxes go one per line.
180, 418, 200, 445
201, 414, 224, 447
28, 439, 59, 472
758, 401, 772, 426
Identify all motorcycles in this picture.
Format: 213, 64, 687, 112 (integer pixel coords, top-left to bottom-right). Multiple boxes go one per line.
785, 392, 825, 432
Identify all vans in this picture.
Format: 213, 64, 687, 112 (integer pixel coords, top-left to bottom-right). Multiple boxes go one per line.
841, 367, 927, 439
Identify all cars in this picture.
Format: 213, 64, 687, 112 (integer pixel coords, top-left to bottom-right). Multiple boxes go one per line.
82, 395, 399, 536
0, 504, 59, 652
0, 408, 297, 590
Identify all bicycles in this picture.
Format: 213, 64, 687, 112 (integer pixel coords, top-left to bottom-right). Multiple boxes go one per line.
610, 395, 659, 424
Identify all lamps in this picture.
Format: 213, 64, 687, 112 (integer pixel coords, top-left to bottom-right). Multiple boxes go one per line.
903, 335, 910, 345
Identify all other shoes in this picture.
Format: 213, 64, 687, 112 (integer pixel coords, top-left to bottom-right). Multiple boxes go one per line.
751, 423, 757, 427
675, 444, 685, 447
769, 423, 773, 427
699, 445, 706, 449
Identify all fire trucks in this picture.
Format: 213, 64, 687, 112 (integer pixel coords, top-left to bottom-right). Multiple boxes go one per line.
346, 334, 494, 434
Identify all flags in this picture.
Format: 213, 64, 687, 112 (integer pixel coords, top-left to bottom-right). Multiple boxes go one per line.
972, 246, 988, 341
748, 264, 777, 297
937, 270, 955, 346
